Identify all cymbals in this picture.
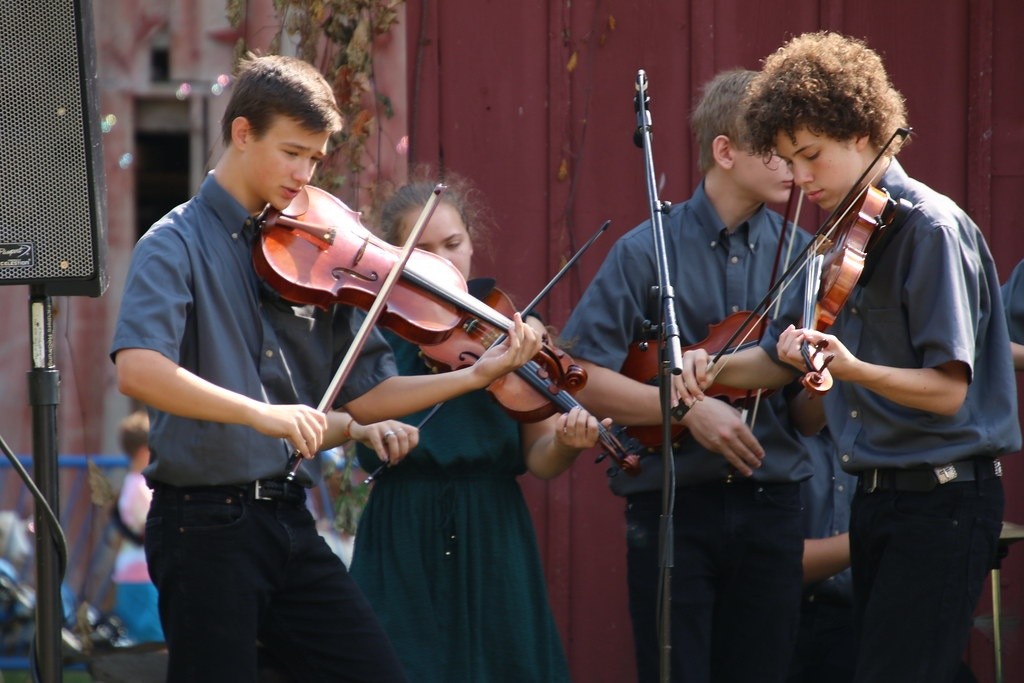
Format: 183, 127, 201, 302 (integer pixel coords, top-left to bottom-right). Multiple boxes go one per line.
1000, 522, 1024, 541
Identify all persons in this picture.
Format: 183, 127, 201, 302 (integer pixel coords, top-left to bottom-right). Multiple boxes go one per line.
109, 27, 1023, 683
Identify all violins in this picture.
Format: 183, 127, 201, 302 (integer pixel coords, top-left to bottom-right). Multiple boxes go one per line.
796, 183, 898, 400
616, 310, 788, 449
251, 183, 591, 396
418, 276, 646, 479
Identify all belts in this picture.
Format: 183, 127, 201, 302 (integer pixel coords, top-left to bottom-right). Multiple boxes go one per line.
153, 478, 307, 510
859, 456, 1008, 494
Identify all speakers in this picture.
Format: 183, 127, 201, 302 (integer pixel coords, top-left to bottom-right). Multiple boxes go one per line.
0, 0, 109, 298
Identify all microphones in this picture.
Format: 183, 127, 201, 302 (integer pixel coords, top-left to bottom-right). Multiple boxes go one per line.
633, 71, 653, 148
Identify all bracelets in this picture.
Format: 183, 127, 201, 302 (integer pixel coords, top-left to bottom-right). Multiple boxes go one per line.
344, 419, 355, 442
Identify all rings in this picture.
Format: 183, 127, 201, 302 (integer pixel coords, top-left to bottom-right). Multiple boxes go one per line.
383, 430, 394, 439
395, 427, 404, 434
